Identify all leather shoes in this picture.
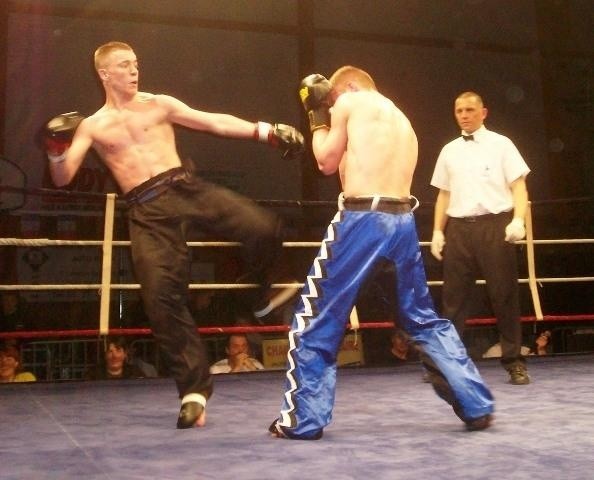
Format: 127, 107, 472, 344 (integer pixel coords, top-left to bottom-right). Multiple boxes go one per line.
508, 365, 529, 386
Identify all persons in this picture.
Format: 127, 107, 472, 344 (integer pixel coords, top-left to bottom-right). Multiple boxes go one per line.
208, 332, 265, 374
525, 328, 553, 355
0, 344, 37, 382
267, 61, 499, 442
422, 89, 532, 388
83, 336, 136, 380
45, 40, 307, 430
372, 329, 420, 367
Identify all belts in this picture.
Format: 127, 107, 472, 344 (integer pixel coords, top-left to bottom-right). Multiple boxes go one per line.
448, 208, 514, 223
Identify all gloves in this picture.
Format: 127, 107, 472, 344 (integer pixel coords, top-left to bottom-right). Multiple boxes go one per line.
39, 107, 85, 164
251, 119, 308, 163
428, 228, 446, 263
503, 217, 525, 244
296, 73, 336, 132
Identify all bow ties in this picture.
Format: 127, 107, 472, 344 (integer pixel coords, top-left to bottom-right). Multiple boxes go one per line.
459, 134, 475, 142
